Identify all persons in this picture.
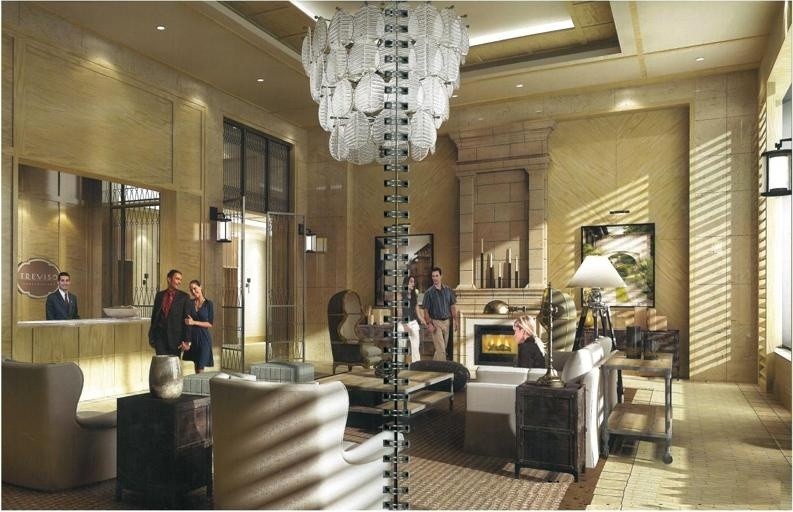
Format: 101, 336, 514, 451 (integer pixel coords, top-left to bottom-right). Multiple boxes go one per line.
421, 266, 459, 362
45, 270, 81, 321
512, 314, 547, 369
146, 268, 193, 361
178, 277, 216, 373
396, 274, 435, 363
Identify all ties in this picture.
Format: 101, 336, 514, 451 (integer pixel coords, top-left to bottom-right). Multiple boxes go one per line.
164, 292, 173, 311
65, 294, 68, 304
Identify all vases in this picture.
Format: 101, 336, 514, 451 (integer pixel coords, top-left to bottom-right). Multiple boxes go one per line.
149, 354, 184, 400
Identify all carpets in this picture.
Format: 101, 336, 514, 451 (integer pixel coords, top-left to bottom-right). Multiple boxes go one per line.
2, 385, 657, 511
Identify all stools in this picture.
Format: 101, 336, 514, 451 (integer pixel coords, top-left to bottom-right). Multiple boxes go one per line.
181, 370, 218, 396
250, 361, 315, 382
411, 359, 471, 392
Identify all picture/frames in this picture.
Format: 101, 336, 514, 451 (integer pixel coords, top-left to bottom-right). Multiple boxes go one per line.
580, 223, 656, 308
374, 233, 434, 308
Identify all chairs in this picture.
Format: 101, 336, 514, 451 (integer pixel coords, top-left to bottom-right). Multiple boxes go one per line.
327, 289, 383, 374
208, 372, 404, 510
2, 358, 118, 494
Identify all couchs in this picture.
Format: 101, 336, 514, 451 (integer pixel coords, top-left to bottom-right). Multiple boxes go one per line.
464, 337, 613, 468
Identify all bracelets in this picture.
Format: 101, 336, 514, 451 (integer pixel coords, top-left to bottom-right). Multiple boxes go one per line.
426, 318, 432, 324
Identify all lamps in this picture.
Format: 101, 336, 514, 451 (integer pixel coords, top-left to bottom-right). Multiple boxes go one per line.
209, 204, 233, 243
565, 254, 624, 404
761, 137, 792, 196
316, 235, 327, 254
297, 222, 319, 255
300, 1, 470, 167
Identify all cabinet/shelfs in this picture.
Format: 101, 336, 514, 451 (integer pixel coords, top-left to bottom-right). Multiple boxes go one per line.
575, 326, 680, 382
515, 380, 586, 483
601, 350, 675, 464
116, 392, 212, 510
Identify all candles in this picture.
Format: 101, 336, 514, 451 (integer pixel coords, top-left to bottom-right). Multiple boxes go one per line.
480, 237, 518, 278
